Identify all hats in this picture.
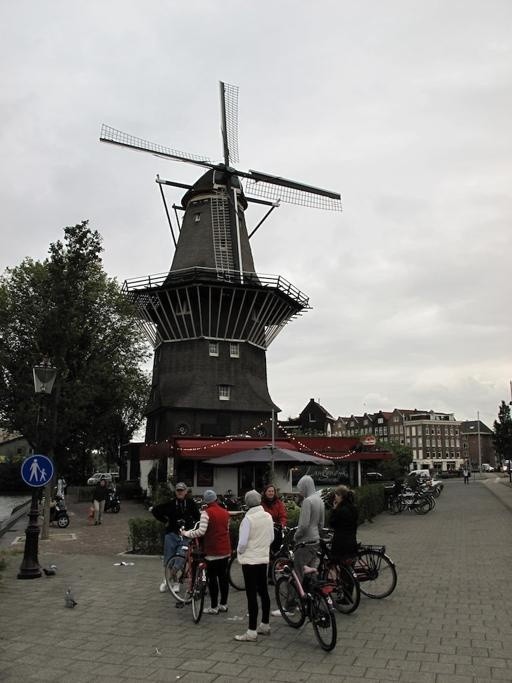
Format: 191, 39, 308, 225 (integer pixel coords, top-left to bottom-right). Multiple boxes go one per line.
482, 458, 512, 472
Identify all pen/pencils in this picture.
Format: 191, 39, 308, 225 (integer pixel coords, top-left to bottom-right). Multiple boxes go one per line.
367, 471, 384, 477
409, 469, 430, 475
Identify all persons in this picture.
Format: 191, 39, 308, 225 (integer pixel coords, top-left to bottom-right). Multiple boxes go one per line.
271, 475, 326, 617
91, 480, 108, 525
263, 484, 287, 585
160, 483, 201, 593
181, 490, 232, 614
234, 490, 275, 641
463, 468, 469, 484
329, 485, 358, 604
39, 476, 67, 526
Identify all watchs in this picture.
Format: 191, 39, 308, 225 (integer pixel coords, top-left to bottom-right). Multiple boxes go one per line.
50, 486, 68, 528
104, 493, 120, 513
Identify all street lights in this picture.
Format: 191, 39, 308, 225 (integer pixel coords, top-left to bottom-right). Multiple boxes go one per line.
89, 507, 94, 518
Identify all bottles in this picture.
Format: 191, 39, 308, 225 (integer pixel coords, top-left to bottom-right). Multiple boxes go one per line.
245, 489, 261, 506
204, 490, 217, 503
176, 482, 186, 490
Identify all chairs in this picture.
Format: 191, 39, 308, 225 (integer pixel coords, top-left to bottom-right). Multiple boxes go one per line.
160, 578, 169, 593
218, 604, 228, 612
271, 607, 294, 617
257, 623, 271, 634
234, 629, 258, 642
335, 596, 353, 606
202, 606, 220, 615
172, 583, 182, 591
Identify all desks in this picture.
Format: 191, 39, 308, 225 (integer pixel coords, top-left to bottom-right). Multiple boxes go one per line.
14, 353, 59, 579
